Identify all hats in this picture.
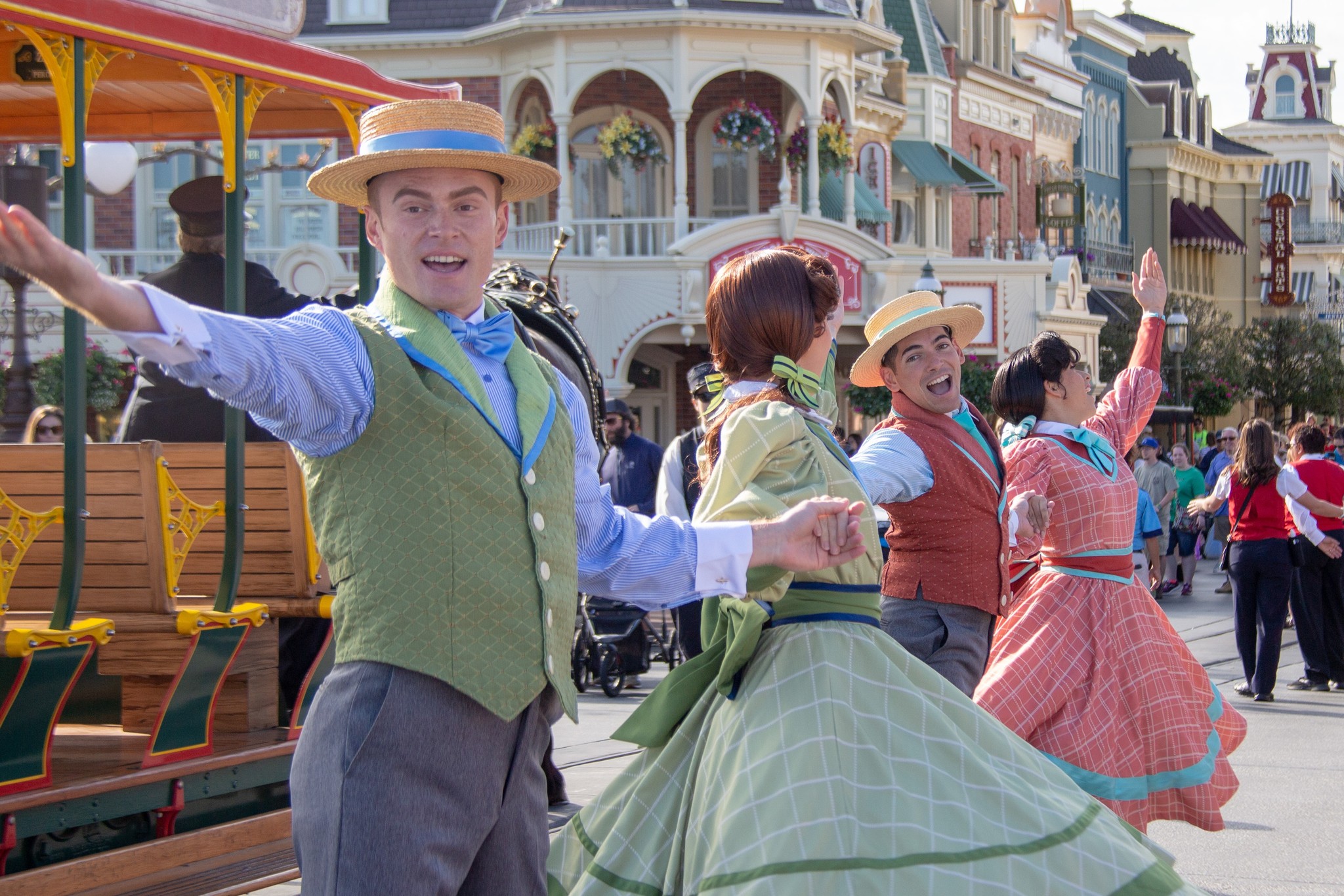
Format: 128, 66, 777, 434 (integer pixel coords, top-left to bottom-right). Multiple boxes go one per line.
1137, 437, 1158, 449
169, 176, 250, 237
601, 398, 636, 428
1215, 430, 1223, 441
307, 101, 562, 207
849, 290, 984, 387
685, 362, 721, 393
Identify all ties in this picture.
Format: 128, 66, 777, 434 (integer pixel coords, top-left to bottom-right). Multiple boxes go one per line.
953, 410, 999, 474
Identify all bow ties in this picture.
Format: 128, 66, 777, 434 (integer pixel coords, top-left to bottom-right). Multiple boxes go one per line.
1065, 428, 1116, 476
436, 310, 515, 366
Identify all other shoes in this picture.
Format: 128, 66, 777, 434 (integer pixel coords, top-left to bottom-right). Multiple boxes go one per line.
1233, 681, 1254, 696
1330, 680, 1344, 690
1215, 580, 1233, 594
1286, 675, 1331, 691
1254, 693, 1274, 701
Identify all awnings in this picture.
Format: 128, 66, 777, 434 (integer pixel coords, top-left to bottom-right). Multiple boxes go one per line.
1171, 198, 1248, 255
892, 140, 1005, 199
801, 159, 893, 225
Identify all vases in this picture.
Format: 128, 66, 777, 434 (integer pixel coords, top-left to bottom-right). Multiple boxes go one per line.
1116, 273, 1128, 281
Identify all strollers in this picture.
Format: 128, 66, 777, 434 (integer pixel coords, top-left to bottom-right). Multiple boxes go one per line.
571, 591, 686, 698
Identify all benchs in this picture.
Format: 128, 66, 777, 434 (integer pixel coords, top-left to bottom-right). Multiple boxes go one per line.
0, 440, 340, 798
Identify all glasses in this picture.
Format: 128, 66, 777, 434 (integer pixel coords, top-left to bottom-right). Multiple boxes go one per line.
1222, 436, 1238, 441
1286, 443, 1302, 449
1334, 445, 1344, 450
605, 415, 623, 425
1217, 440, 1222, 443
693, 391, 721, 402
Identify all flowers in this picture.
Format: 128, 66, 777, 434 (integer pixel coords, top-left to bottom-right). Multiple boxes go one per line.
34, 339, 138, 414
512, 116, 576, 176
1060, 248, 1094, 265
594, 109, 673, 181
1156, 390, 1174, 406
781, 117, 856, 180
1190, 379, 1240, 417
841, 378, 892, 417
710, 97, 780, 165
959, 356, 1003, 415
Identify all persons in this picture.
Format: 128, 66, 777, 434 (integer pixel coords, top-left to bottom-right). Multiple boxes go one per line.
548, 246, 1192, 896
831, 426, 862, 459
1162, 418, 1239, 596
114, 177, 359, 442
26, 405, 64, 443
1187, 415, 1344, 700
1122, 442, 1163, 591
600, 398, 664, 517
655, 363, 724, 661
974, 247, 1247, 836
847, 290, 1055, 700
1133, 436, 1177, 599
0, 99, 866, 896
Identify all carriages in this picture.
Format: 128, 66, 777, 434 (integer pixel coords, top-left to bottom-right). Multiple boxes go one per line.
0, 0, 607, 896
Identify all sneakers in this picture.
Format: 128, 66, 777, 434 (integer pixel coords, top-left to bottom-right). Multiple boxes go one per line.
1180, 582, 1193, 595
1162, 580, 1179, 595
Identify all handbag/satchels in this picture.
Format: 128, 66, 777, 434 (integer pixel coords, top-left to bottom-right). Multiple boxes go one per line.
1172, 506, 1197, 533
1219, 541, 1231, 570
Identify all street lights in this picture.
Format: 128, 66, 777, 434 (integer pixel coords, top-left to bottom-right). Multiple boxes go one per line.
0, 137, 140, 444
1165, 304, 1190, 442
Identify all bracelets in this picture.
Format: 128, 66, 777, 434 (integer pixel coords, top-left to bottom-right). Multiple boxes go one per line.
1338, 507, 1344, 518
1156, 504, 1162, 511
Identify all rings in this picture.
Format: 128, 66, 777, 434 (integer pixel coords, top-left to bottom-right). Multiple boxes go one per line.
1329, 550, 1331, 553
